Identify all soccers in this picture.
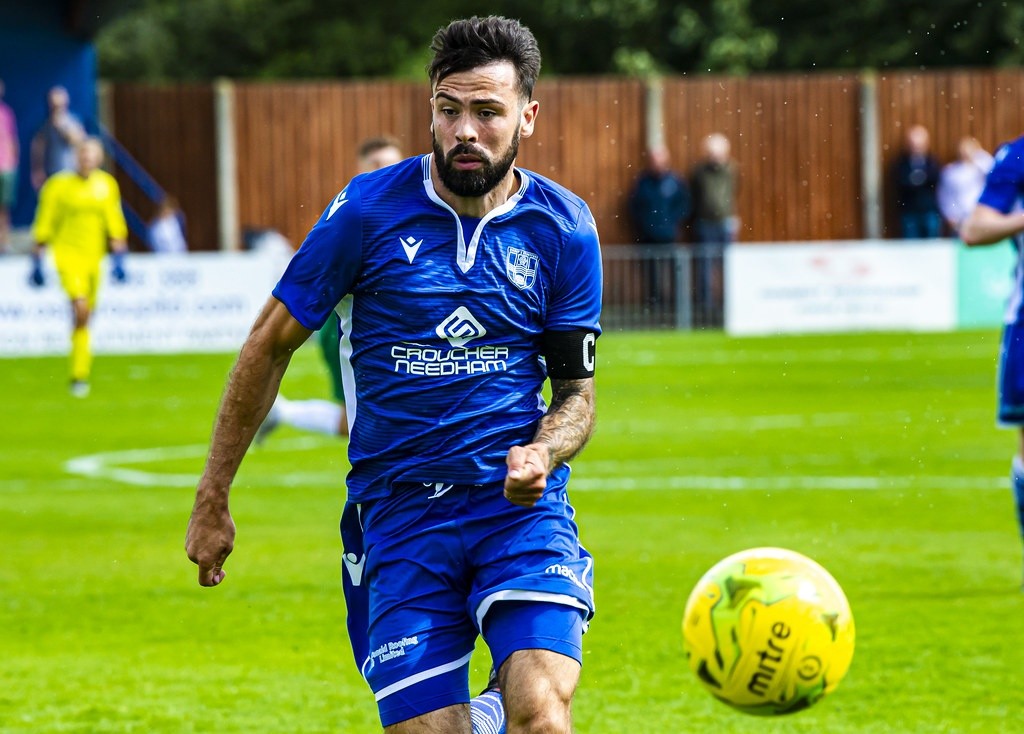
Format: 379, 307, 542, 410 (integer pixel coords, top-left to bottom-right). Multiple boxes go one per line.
683, 547, 856, 716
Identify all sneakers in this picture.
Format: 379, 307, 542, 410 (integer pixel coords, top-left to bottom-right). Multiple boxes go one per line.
480, 663, 498, 695
72, 381, 90, 399
256, 412, 276, 444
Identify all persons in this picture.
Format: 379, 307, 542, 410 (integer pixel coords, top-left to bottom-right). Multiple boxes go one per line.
622, 142, 693, 311
687, 131, 744, 307
961, 135, 1023, 543
0, 80, 19, 255
891, 122, 997, 239
28, 84, 89, 192
320, 139, 405, 434
26, 133, 130, 398
185, 13, 600, 733
